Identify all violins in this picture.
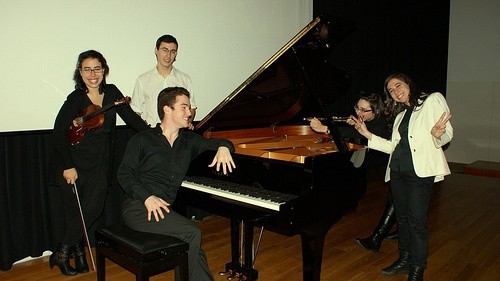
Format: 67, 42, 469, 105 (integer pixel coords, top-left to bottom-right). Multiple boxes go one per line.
65, 95, 131, 146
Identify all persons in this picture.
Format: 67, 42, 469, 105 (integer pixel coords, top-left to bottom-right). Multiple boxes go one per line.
45, 49, 153, 276
115, 87, 237, 281
309, 93, 451, 253
128, 34, 198, 131
351, 74, 454, 281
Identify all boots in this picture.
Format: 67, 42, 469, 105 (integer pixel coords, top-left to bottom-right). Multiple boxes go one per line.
353, 199, 395, 253
380, 247, 412, 275
49, 243, 77, 276
385, 230, 399, 240
408, 266, 425, 281
73, 242, 90, 273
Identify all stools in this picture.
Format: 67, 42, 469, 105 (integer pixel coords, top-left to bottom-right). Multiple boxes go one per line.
94, 225, 190, 281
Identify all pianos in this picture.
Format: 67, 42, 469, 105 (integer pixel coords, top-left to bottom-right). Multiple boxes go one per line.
179, 16, 396, 281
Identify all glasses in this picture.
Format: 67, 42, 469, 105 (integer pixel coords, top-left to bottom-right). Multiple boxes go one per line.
82, 67, 104, 74
354, 105, 373, 113
